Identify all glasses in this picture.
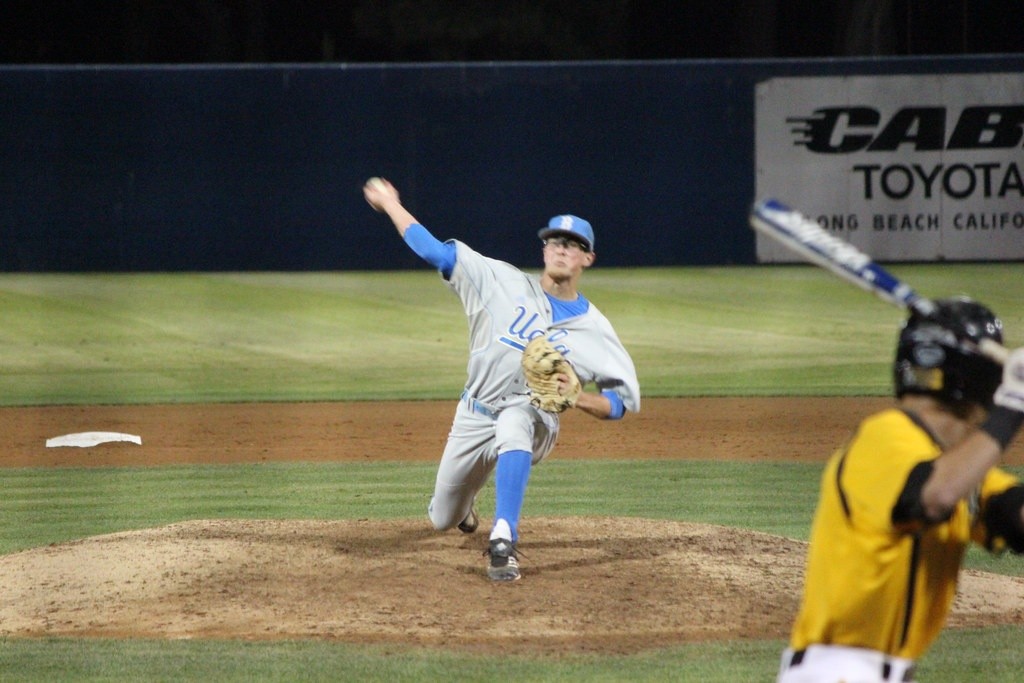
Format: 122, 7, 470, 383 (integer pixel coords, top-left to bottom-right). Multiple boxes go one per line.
543, 238, 587, 253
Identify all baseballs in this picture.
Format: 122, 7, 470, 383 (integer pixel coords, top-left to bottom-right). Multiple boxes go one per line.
365, 176, 387, 193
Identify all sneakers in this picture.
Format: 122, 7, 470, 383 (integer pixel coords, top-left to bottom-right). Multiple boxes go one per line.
482, 538, 527, 583
457, 509, 478, 534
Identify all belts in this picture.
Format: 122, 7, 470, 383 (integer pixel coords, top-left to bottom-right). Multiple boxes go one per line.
790, 650, 890, 681
461, 388, 501, 420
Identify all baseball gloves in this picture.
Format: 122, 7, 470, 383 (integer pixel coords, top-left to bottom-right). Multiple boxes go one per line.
520, 335, 582, 413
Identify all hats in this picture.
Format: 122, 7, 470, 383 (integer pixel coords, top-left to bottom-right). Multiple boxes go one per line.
538, 214, 595, 253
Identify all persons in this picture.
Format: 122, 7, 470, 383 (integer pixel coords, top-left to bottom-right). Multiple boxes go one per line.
363, 176, 642, 584
776, 300, 1024, 682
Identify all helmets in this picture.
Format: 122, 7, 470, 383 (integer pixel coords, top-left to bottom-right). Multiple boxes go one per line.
893, 299, 1004, 413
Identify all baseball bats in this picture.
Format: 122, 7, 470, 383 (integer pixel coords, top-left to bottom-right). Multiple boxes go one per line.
748, 195, 1015, 369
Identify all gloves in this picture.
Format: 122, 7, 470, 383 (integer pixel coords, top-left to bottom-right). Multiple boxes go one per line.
981, 348, 1024, 450
